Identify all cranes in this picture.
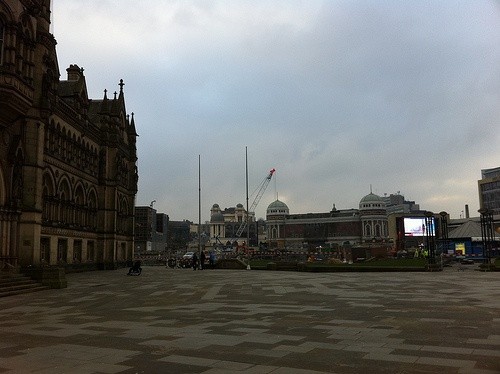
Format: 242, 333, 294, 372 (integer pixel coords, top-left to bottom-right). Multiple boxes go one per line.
232, 168, 276, 244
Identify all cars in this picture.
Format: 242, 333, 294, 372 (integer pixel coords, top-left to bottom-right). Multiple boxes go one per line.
183, 252, 194, 259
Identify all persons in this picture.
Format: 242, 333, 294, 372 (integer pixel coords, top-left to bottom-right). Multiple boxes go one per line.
414, 247, 428, 258
191, 253, 197, 271
200, 251, 205, 270
209, 252, 214, 265
